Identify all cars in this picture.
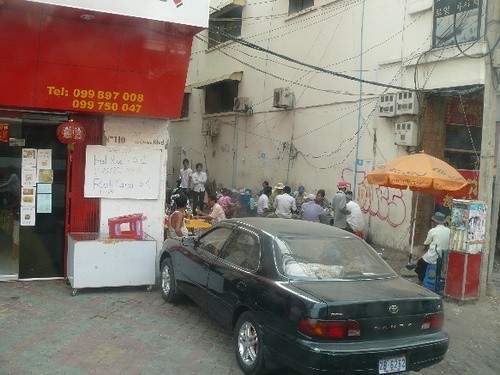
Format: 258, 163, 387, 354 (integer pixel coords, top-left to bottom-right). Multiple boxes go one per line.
158, 216, 450, 375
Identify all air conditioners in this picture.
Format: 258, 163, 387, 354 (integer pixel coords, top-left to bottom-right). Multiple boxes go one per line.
273, 87, 291, 108
233, 96, 250, 113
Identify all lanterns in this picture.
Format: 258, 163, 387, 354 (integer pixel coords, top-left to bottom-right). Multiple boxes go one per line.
57, 119, 87, 164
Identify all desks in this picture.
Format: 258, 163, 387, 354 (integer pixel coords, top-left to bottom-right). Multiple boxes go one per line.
66, 232, 157, 296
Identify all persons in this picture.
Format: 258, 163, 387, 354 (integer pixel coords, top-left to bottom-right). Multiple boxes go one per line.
168, 195, 190, 239
177, 159, 193, 193
167, 192, 195, 218
330, 180, 352, 230
346, 189, 366, 235
257, 187, 278, 217
274, 185, 301, 220
190, 162, 208, 216
270, 182, 287, 206
193, 194, 229, 224
219, 186, 258, 219
312, 189, 333, 212
299, 194, 333, 223
293, 184, 311, 211
407, 211, 452, 285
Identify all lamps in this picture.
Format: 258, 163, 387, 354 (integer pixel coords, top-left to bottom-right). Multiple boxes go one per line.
202, 119, 220, 136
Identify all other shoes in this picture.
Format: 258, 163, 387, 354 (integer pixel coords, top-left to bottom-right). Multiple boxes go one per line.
405, 264, 416, 271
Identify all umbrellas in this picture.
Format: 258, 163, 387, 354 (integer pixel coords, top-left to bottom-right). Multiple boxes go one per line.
369, 150, 469, 268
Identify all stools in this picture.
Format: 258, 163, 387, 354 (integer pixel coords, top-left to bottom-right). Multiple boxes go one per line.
422, 263, 445, 291
108, 213, 147, 239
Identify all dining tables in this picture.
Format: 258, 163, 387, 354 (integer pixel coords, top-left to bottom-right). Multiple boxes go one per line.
164, 214, 211, 239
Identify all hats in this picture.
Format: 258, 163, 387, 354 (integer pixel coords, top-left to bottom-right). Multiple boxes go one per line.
337, 181, 348, 188
275, 183, 285, 190
431, 212, 446, 224
297, 186, 305, 192
